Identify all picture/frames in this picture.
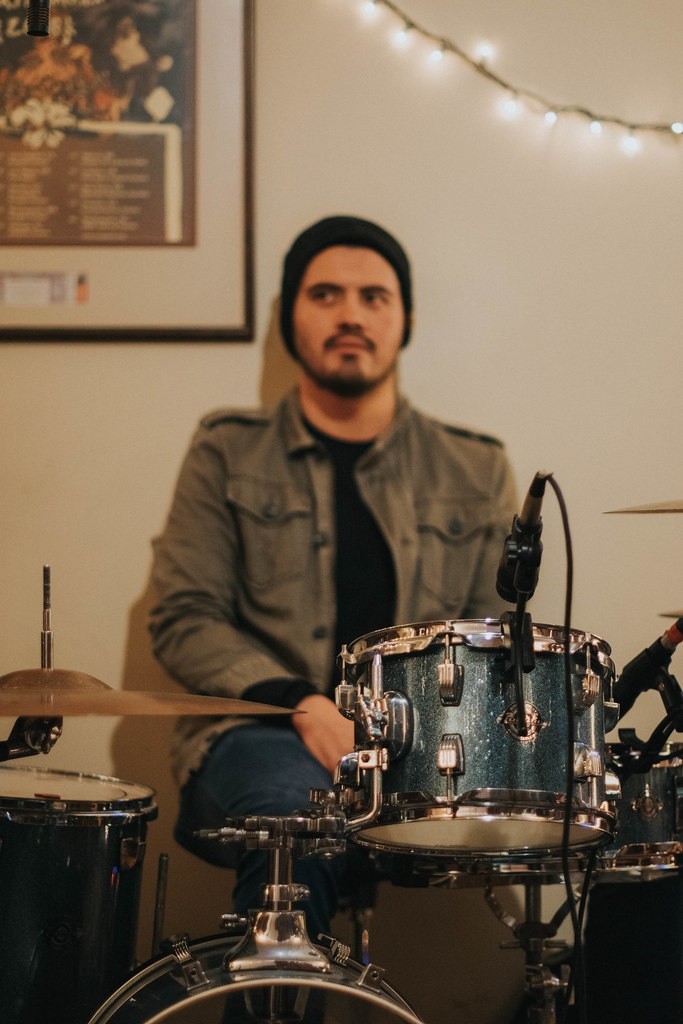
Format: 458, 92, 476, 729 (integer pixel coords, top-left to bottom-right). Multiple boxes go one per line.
0, 1, 256, 341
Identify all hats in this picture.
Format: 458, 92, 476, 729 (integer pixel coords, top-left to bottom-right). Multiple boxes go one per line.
281, 216, 413, 361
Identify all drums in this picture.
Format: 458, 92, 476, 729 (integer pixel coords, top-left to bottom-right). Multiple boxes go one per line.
315, 617, 621, 872
0, 765, 158, 1024
88, 929, 422, 1023
578, 742, 683, 885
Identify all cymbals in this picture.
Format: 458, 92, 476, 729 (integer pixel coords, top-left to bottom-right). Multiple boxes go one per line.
1, 669, 311, 716
603, 500, 683, 514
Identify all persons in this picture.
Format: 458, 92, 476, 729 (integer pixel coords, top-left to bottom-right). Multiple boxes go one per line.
149, 213, 521, 1023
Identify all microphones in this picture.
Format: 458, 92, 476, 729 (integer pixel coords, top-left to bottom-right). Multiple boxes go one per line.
613, 618, 683, 717
497, 471, 547, 603
